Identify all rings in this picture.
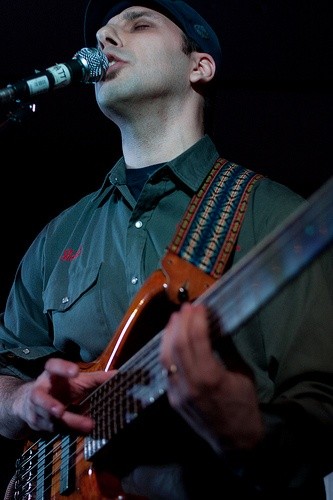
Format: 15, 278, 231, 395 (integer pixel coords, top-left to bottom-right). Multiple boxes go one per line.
163, 363, 176, 378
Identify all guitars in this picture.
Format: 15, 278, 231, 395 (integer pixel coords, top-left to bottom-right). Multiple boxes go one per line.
4, 178, 333, 500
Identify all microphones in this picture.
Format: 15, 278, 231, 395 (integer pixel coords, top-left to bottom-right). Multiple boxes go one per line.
5, 47, 109, 106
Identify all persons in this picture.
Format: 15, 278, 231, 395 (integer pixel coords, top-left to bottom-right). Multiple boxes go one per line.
1, 2, 333, 499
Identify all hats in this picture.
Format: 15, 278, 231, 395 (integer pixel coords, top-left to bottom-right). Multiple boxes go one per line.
83, 0, 220, 60
